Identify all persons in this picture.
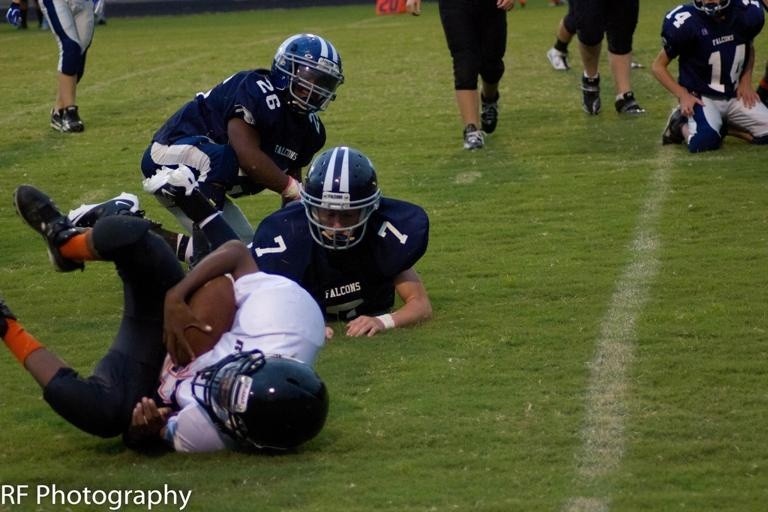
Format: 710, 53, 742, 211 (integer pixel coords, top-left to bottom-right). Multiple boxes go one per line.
0, 184, 332, 458
546, 0, 575, 71
576, 0, 644, 117
6, 0, 105, 133
406, 0, 514, 150
65, 32, 345, 267
652, 0, 768, 155
141, 145, 433, 342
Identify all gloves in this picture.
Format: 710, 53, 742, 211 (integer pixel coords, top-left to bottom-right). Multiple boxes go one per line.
5, 2, 22, 27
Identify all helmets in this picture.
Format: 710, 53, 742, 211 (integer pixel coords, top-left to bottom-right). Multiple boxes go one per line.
695, 0, 731, 14
299, 146, 382, 250
270, 33, 344, 116
190, 349, 328, 451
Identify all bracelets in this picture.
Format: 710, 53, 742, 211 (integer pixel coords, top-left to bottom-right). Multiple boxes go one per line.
376, 313, 395, 329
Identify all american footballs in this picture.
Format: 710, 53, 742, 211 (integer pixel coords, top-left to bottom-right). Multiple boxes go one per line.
177, 275, 237, 367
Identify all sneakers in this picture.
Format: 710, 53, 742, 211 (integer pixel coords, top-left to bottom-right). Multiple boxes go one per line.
13, 183, 85, 274
50, 105, 84, 132
0, 298, 17, 339
142, 163, 199, 197
67, 191, 145, 227
464, 47, 689, 150
